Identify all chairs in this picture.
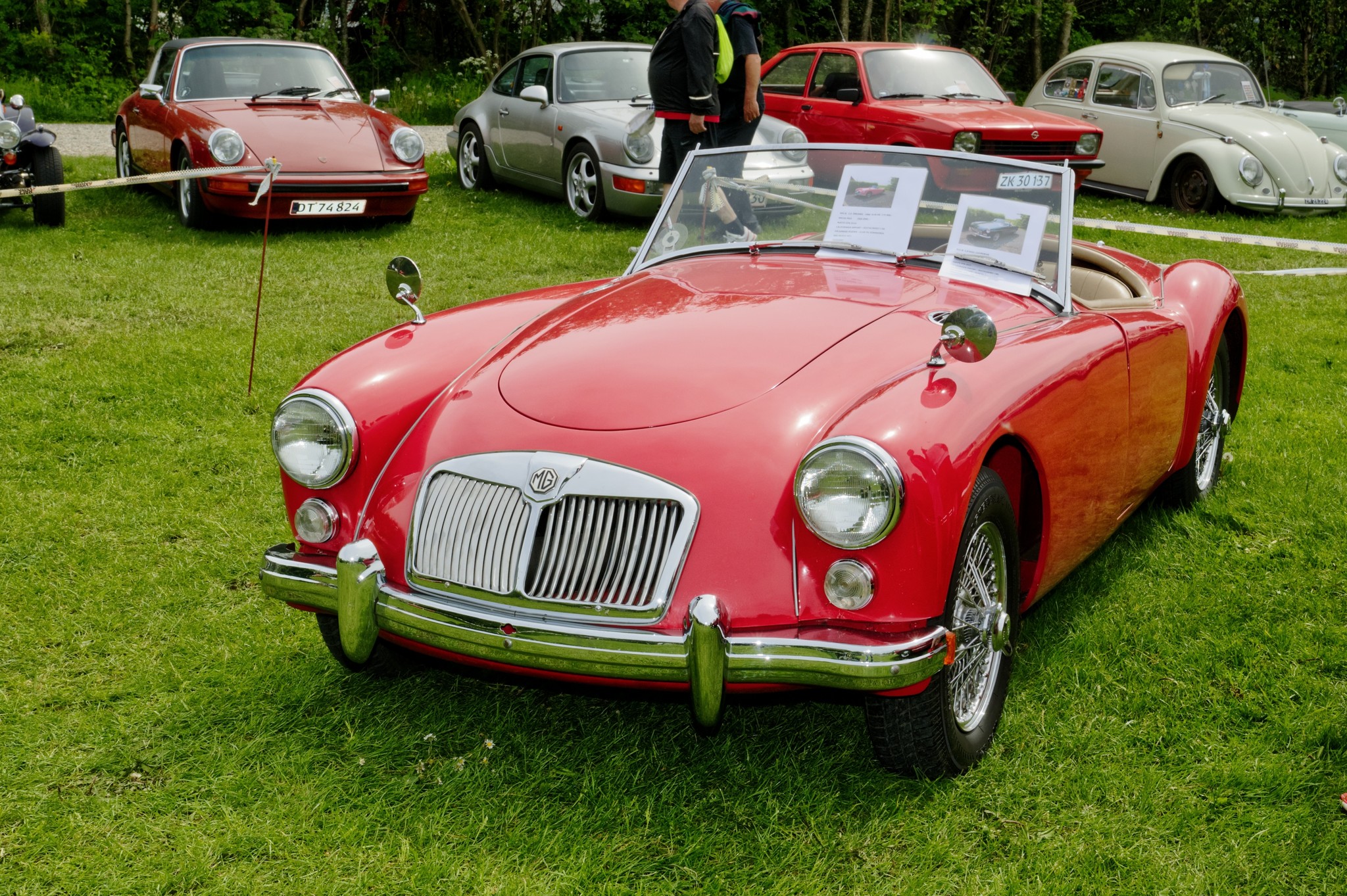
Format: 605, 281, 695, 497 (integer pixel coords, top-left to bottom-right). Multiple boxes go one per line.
817, 72, 858, 98
250, 65, 295, 96
178, 59, 231, 99
534, 68, 576, 101
932, 243, 1134, 300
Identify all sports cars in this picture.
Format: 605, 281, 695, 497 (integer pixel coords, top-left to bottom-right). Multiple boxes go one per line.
446, 42, 815, 222
969, 218, 1019, 242
256, 141, 1250, 784
111, 36, 430, 229
855, 185, 885, 198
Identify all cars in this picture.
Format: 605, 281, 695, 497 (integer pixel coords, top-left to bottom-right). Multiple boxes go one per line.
760, 42, 1106, 215
0, 87, 66, 228
1022, 41, 1347, 218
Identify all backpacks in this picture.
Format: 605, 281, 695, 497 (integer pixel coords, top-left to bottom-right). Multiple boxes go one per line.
713, 13, 734, 85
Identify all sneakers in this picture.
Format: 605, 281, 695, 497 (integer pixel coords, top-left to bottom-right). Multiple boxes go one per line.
628, 242, 674, 255
723, 225, 758, 243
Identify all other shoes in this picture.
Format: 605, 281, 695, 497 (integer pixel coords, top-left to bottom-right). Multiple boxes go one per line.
697, 220, 762, 242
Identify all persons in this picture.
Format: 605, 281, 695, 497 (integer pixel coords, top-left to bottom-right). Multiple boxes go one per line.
708, 0, 764, 240
630, 0, 756, 255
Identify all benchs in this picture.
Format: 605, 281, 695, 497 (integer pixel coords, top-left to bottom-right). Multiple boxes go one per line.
1083, 92, 1138, 105
908, 224, 1152, 297
